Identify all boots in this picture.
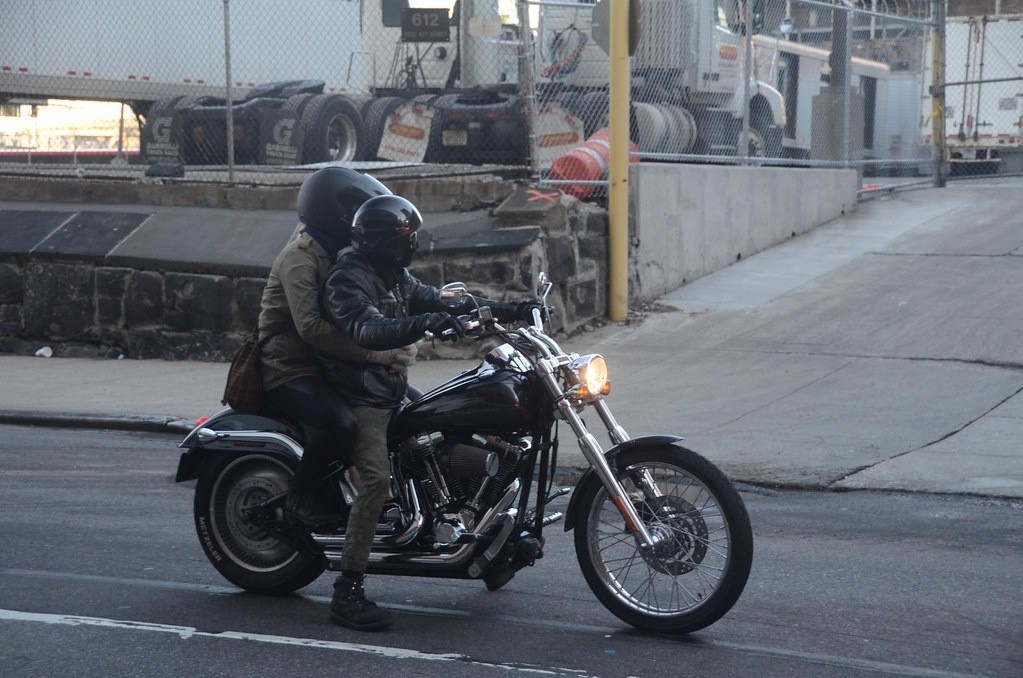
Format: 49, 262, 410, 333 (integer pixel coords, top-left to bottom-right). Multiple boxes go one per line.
484, 537, 546, 592
329, 574, 393, 631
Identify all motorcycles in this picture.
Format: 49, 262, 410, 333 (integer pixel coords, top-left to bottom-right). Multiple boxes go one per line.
175, 272, 754, 634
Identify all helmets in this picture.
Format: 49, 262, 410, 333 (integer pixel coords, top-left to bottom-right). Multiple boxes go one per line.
296, 166, 394, 252
350, 195, 424, 267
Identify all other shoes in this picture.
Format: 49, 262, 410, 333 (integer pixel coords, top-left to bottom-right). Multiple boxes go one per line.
285, 479, 345, 529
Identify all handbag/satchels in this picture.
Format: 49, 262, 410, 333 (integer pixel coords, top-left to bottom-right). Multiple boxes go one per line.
222, 334, 274, 415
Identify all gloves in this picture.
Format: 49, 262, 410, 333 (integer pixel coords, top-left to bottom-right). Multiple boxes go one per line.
422, 311, 465, 342
516, 300, 553, 325
361, 343, 419, 373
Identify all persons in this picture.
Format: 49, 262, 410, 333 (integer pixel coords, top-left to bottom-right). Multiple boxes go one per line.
318, 196, 554, 627
258, 167, 428, 531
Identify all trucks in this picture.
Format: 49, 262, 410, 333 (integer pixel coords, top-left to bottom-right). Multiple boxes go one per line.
536, 1, 788, 171
913, 12, 1022, 172
